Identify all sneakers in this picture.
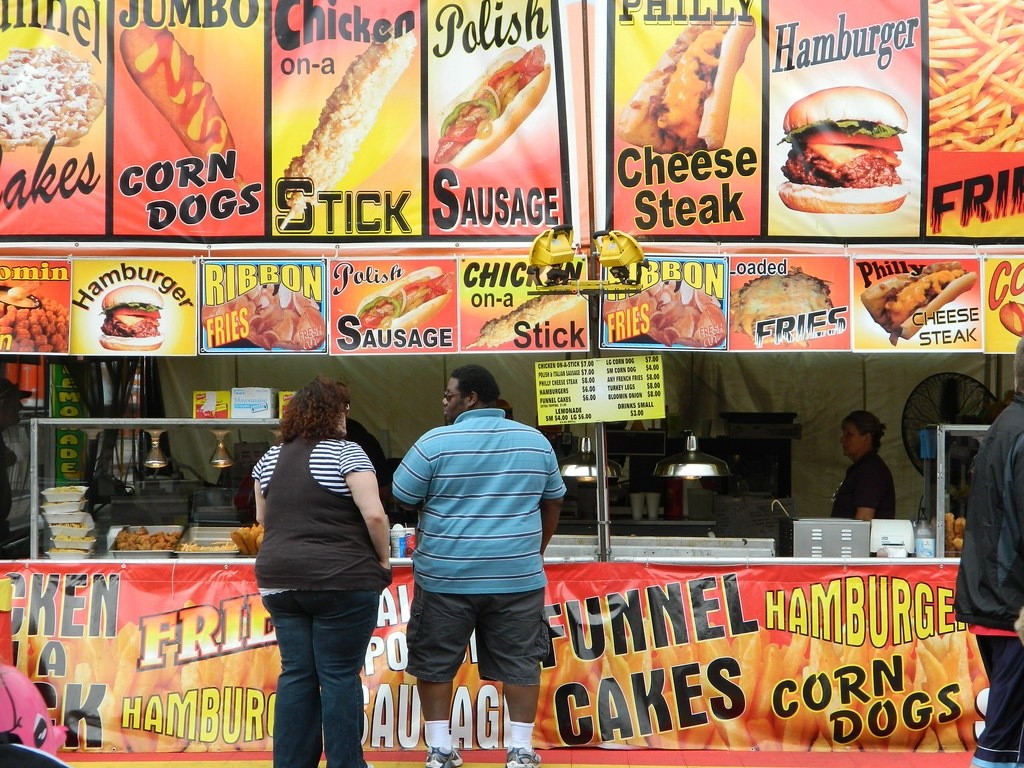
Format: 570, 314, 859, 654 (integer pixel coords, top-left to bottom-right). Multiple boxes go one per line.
505, 746, 542, 768
425, 745, 463, 768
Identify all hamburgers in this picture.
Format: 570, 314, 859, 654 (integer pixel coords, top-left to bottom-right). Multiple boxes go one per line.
101, 285, 165, 352
778, 87, 912, 213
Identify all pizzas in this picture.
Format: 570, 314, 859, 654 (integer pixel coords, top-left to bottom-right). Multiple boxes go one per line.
0, 48, 104, 155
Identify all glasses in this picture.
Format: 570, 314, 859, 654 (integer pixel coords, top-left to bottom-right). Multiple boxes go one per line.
443, 392, 471, 402
345, 403, 350, 411
830, 480, 844, 503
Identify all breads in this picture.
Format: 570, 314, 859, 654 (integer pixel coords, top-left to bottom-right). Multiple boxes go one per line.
121, 24, 236, 169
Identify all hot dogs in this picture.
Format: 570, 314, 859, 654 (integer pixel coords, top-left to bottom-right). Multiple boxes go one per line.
357, 266, 451, 344
620, 21, 757, 151
861, 261, 978, 346
432, 46, 550, 169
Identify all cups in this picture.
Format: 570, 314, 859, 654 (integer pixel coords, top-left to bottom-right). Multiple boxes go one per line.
404, 528, 416, 557
646, 493, 660, 520
629, 493, 645, 521
390, 530, 406, 559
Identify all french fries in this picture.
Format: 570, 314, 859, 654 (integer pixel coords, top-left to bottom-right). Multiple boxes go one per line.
30, 613, 980, 753
926, 1, 1024, 155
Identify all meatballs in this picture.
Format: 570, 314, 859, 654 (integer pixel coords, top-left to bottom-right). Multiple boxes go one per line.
0, 293, 69, 352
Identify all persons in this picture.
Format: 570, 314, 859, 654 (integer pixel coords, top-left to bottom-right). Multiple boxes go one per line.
954, 338, 1024, 768
252, 376, 393, 768
393, 365, 568, 768
830, 410, 896, 521
